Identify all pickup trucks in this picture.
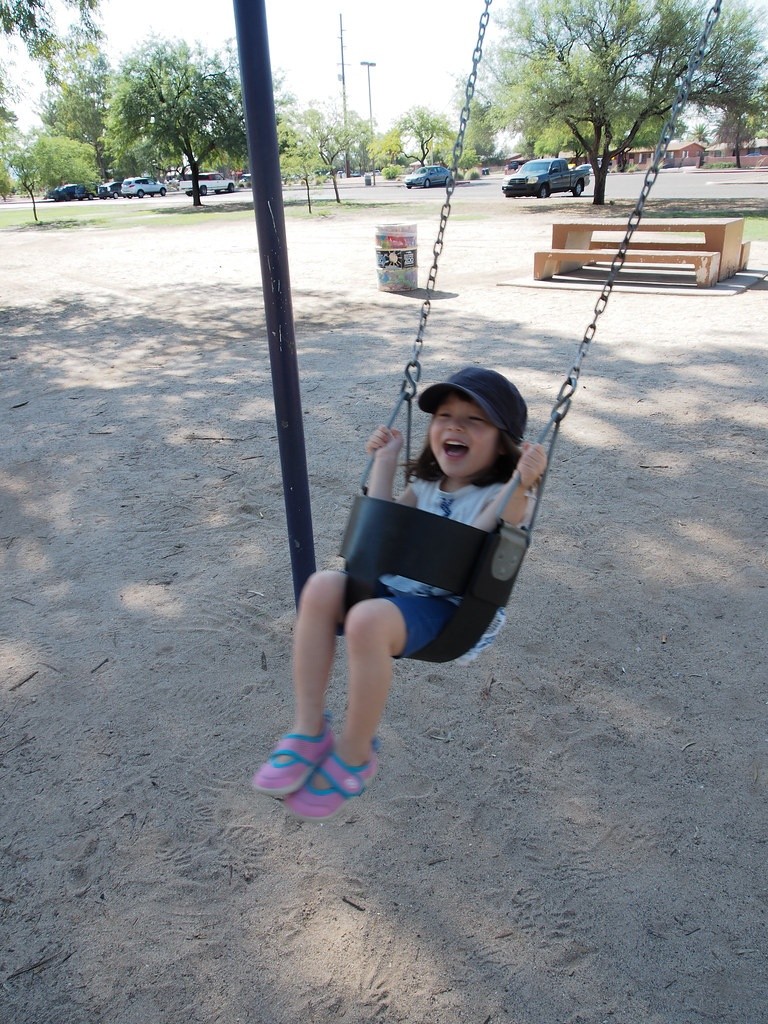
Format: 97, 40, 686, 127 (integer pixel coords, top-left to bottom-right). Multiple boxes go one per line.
502, 158, 592, 198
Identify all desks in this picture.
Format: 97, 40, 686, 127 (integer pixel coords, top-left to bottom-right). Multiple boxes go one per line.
552, 218, 745, 282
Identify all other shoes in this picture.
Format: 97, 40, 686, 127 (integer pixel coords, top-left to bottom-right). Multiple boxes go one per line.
252, 710, 336, 796
283, 737, 381, 822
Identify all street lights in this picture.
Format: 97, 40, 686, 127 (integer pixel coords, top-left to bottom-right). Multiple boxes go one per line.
361, 62, 376, 185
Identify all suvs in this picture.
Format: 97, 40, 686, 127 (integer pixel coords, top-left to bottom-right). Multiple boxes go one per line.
75, 182, 99, 201
121, 177, 166, 199
179, 172, 234, 196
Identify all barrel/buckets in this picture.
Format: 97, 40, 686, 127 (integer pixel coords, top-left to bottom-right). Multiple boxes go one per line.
374, 220, 419, 292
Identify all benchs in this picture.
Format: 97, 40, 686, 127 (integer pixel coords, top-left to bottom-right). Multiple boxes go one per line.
584, 240, 751, 274
533, 248, 721, 288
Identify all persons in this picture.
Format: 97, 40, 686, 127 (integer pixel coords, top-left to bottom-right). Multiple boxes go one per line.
249, 367, 548, 822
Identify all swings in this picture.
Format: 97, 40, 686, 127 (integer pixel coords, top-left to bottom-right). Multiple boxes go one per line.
337, 0, 724, 665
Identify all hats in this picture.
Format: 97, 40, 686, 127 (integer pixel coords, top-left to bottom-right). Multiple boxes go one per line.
417, 367, 527, 442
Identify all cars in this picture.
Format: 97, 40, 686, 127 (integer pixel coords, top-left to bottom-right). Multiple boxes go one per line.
405, 166, 453, 189
98, 182, 132, 200
349, 172, 360, 177
49, 184, 78, 201
